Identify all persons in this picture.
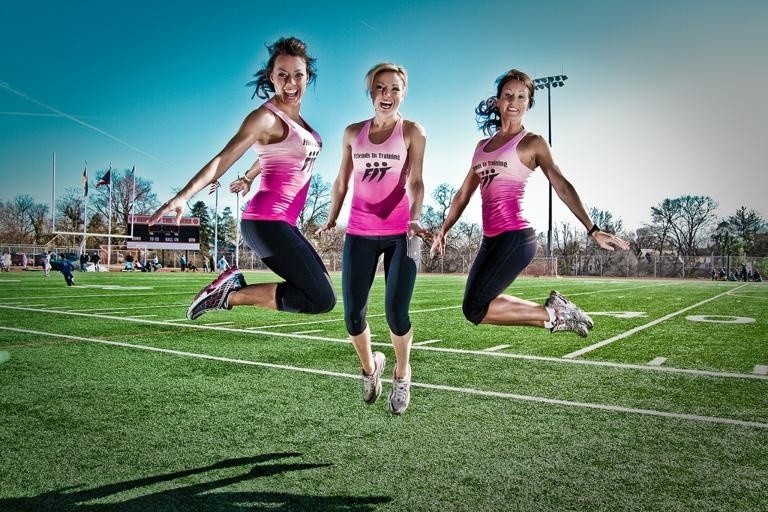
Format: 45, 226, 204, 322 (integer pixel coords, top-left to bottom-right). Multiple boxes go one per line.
125, 251, 230, 275
429, 69, 632, 338
57, 255, 76, 286
0, 250, 28, 272
89, 253, 101, 272
314, 62, 434, 416
712, 263, 762, 282
145, 37, 337, 321
43, 251, 52, 277
79, 250, 89, 273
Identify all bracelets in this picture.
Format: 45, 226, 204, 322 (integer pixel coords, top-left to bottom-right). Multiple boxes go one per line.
244, 169, 255, 182
407, 219, 420, 224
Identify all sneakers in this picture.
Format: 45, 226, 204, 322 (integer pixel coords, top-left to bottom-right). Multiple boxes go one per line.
362, 351, 386, 404
388, 363, 411, 414
186, 265, 247, 321
545, 290, 594, 338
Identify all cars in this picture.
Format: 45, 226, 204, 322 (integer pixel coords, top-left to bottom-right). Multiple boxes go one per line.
12, 252, 125, 266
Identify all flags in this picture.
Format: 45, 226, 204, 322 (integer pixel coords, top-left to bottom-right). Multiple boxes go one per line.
81, 167, 89, 197
95, 168, 111, 188
208, 179, 221, 195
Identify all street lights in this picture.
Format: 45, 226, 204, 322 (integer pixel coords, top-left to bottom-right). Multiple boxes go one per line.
533, 74, 569, 279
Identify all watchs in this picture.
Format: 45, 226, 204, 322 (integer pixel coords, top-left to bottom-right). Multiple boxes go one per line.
587, 224, 601, 237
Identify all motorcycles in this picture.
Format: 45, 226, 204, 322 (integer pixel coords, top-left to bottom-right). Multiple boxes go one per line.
712, 268, 762, 282
